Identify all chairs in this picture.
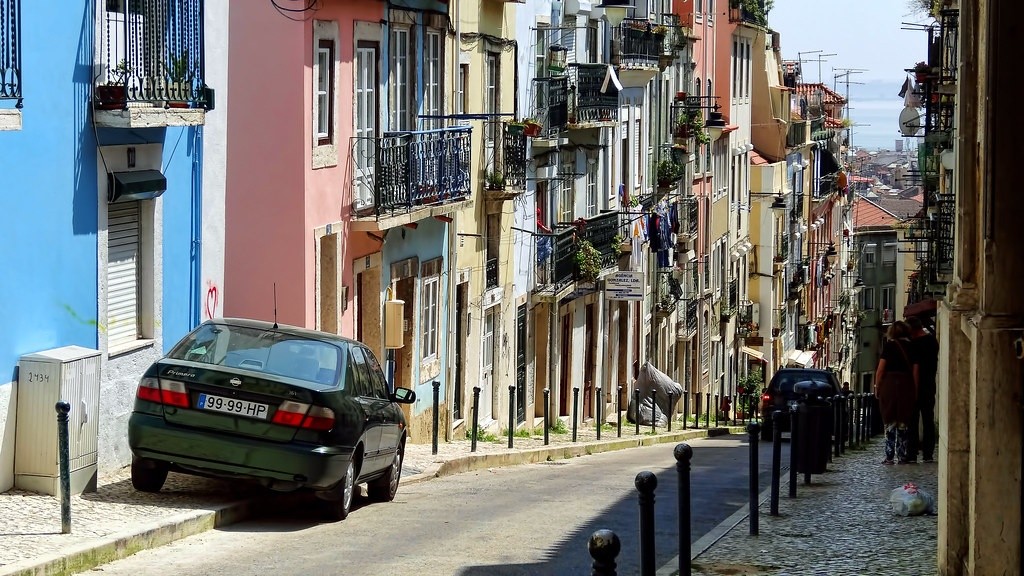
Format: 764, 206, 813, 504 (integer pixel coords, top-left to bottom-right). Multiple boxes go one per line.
289, 358, 320, 380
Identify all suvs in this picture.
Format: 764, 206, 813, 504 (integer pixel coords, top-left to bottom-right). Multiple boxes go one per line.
760, 366, 850, 441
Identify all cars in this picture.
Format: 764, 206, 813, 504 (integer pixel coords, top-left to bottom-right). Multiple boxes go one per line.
126, 317, 417, 520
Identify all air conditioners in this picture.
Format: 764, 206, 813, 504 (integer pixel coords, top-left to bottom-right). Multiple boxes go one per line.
803, 266, 809, 283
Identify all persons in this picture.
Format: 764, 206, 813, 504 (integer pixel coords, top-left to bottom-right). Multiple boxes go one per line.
843, 382, 854, 396
873, 320, 940, 464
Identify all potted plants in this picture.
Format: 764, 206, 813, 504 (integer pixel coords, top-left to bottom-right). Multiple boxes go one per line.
97, 60, 136, 111
158, 52, 198, 106
915, 62, 930, 82
627, 22, 667, 43
719, 368, 762, 420
677, 104, 710, 146
523, 116, 544, 138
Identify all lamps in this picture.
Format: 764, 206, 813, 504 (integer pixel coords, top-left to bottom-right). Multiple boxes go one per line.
108, 170, 168, 204
842, 274, 866, 292
808, 240, 838, 263
750, 189, 790, 218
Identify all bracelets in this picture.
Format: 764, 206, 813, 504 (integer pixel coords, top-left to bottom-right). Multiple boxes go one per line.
874, 385, 879, 387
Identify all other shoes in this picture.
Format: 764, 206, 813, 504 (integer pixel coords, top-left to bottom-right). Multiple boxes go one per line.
923, 457, 933, 462
879, 456, 895, 465
898, 457, 906, 464
906, 455, 917, 462
